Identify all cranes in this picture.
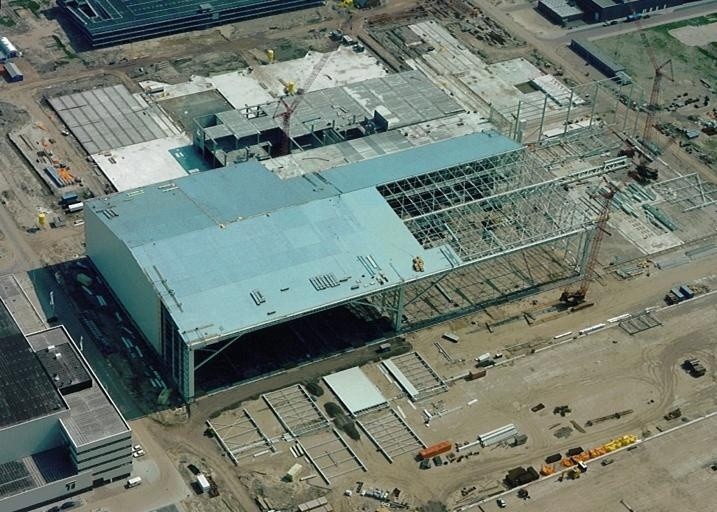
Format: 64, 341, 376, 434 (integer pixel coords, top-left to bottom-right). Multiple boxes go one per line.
268, 12, 356, 155
621, 0, 679, 183
556, 126, 694, 309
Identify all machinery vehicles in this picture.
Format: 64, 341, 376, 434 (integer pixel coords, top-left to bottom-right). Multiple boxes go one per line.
559, 461, 587, 482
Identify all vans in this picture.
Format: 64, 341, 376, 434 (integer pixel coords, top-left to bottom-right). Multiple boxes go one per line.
127, 476, 142, 488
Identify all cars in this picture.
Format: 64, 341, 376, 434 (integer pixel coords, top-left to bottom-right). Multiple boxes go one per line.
602, 454, 613, 466
130, 444, 145, 458
496, 497, 505, 508
